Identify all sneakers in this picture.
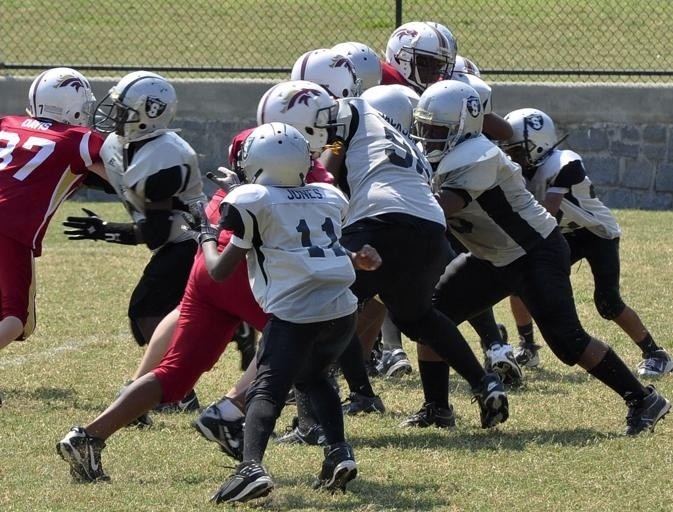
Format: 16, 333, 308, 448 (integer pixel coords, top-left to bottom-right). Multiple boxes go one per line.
271, 417, 329, 446
310, 443, 358, 495
397, 404, 456, 430
195, 398, 246, 464
285, 373, 340, 405
624, 385, 671, 435
637, 345, 673, 380
56, 426, 109, 482
125, 412, 155, 430
233, 322, 259, 371
471, 374, 511, 430
480, 324, 507, 371
341, 392, 385, 415
207, 459, 274, 505
326, 336, 413, 378
152, 389, 199, 415
515, 342, 540, 367
486, 342, 521, 389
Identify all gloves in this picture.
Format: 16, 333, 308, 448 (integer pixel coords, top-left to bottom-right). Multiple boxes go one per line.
180, 200, 222, 246
207, 167, 242, 194
62, 209, 136, 246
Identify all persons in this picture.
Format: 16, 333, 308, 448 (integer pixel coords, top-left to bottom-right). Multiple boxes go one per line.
1, 67, 110, 350
62, 71, 258, 414
56, 81, 340, 482
126, 21, 673, 505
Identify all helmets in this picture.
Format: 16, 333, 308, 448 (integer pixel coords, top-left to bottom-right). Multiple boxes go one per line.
427, 20, 484, 114
413, 79, 484, 163
93, 70, 177, 140
28, 66, 97, 127
291, 49, 357, 99
332, 41, 382, 92
361, 84, 412, 136
494, 109, 560, 172
241, 122, 311, 188
385, 22, 455, 92
256, 80, 348, 161
